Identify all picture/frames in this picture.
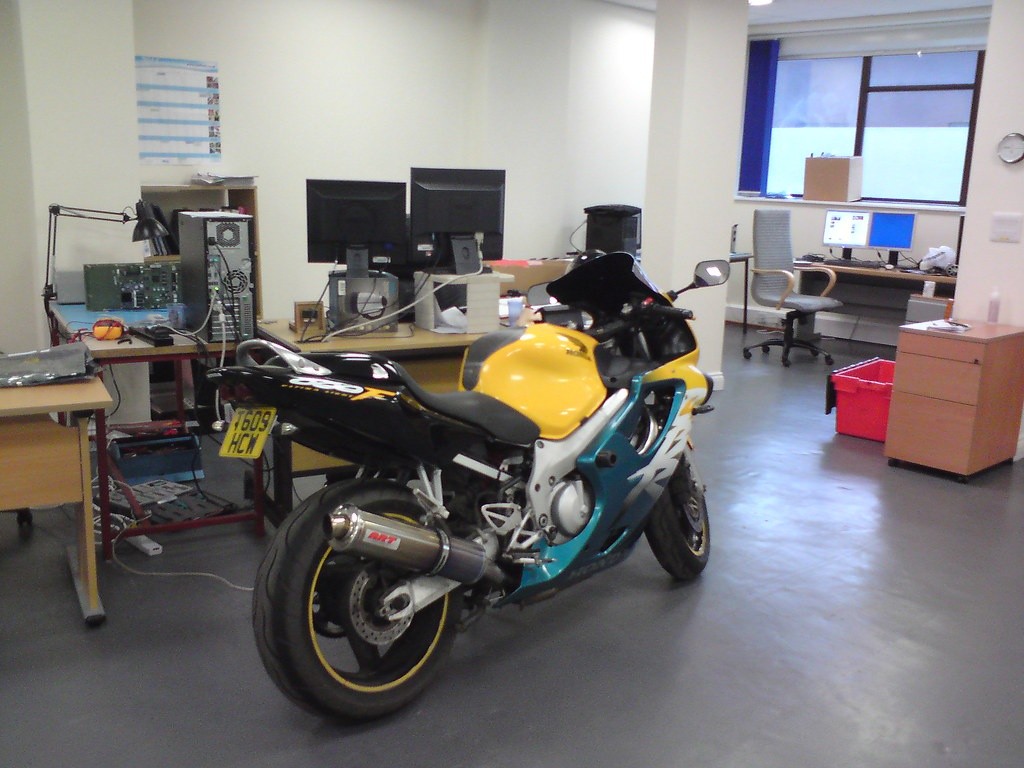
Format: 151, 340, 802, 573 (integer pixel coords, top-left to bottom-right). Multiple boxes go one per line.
295, 300, 327, 334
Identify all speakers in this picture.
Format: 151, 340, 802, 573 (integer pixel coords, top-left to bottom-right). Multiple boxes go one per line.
586, 214, 638, 257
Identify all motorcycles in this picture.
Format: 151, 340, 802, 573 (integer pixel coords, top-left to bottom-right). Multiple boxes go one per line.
203, 251, 730, 727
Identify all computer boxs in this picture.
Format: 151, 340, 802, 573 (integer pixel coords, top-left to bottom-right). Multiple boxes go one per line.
178, 211, 256, 342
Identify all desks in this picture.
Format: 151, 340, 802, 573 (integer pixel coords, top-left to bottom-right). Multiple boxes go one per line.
793, 261, 957, 344
50, 303, 263, 565
0, 372, 113, 629
730, 250, 754, 334
254, 319, 510, 533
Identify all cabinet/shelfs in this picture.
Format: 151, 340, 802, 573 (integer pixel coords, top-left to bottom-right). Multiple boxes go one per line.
883, 318, 1024, 484
140, 185, 263, 319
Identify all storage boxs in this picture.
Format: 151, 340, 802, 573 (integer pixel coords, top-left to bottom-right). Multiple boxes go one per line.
803, 155, 863, 202
825, 356, 896, 443
480, 258, 572, 297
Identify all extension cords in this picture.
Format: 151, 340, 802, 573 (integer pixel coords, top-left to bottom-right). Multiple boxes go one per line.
129, 326, 175, 347
124, 535, 162, 555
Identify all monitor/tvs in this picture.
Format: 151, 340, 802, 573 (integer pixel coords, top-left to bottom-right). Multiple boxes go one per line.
823, 209, 916, 266
306, 167, 507, 276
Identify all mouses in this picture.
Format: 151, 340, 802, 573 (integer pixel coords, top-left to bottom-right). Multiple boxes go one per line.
885, 264, 894, 270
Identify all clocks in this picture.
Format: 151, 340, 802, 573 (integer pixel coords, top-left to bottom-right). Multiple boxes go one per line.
998, 133, 1024, 164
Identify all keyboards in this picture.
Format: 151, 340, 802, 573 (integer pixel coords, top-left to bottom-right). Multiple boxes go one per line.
825, 259, 885, 268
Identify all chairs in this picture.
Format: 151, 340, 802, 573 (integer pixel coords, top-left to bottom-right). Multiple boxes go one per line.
742, 209, 844, 368
